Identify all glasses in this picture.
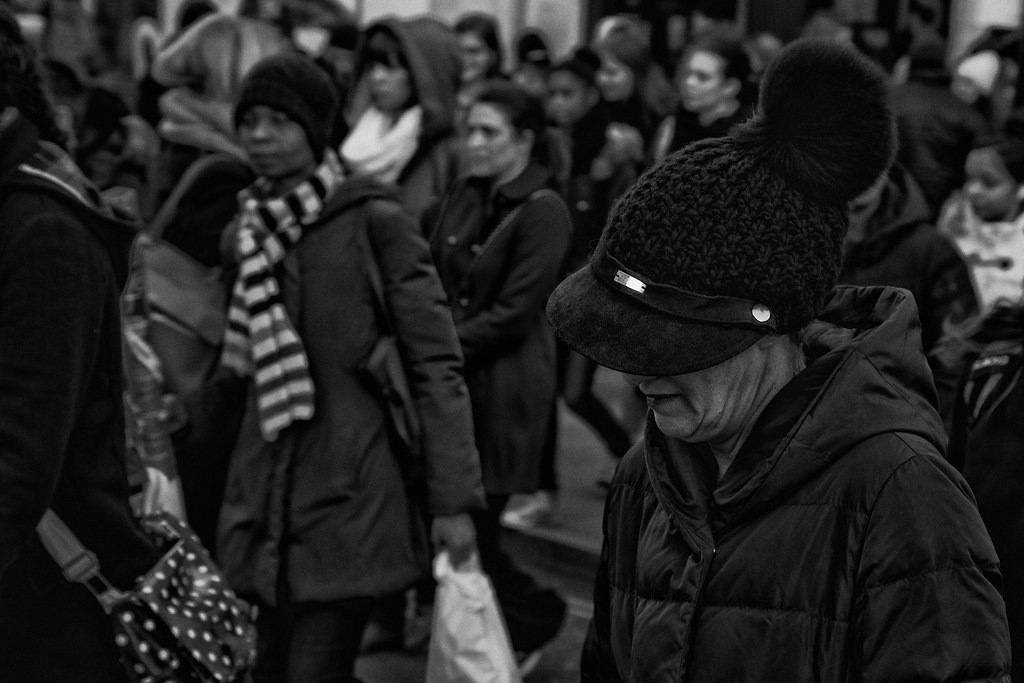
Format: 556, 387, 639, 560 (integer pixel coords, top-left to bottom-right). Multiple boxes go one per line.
528, 53, 552, 66
458, 46, 490, 55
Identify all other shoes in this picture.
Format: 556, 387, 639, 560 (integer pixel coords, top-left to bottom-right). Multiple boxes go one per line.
511, 643, 543, 676
598, 455, 623, 488
358, 607, 430, 657
502, 491, 572, 529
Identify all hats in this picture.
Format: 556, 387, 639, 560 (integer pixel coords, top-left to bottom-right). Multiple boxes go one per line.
957, 49, 1000, 95
548, 35, 897, 381
518, 32, 545, 63
231, 54, 339, 164
600, 16, 637, 45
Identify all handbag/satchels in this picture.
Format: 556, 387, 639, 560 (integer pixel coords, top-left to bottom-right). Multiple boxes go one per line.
425, 547, 522, 683
356, 195, 424, 460
126, 151, 260, 443
117, 511, 258, 683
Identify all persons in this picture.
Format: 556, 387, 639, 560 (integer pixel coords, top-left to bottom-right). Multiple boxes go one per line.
0, 8, 130, 683
544, 43, 1012, 682
41, 1, 1024, 682
